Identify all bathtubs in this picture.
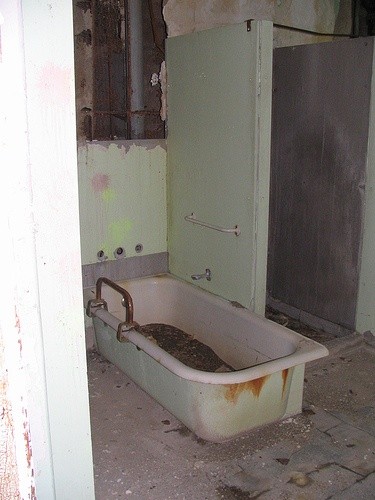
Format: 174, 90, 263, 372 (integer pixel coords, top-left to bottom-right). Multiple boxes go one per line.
83, 271, 329, 445
